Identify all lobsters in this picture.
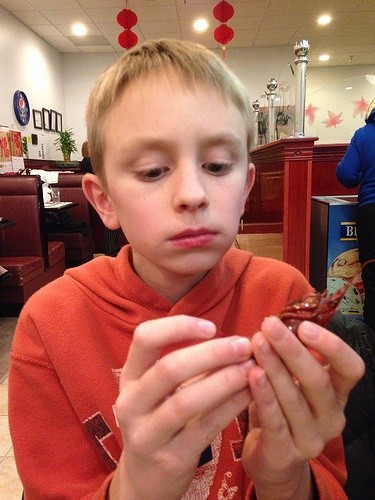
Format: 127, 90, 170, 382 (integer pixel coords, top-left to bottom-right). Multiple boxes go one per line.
277, 259, 375, 351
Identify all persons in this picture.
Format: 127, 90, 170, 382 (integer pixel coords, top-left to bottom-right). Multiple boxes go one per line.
335, 108, 375, 332
8, 38, 365, 500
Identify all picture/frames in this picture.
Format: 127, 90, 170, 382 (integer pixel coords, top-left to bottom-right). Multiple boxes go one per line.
13, 90, 30, 126
33, 110, 42, 129
42, 108, 50, 131
50, 109, 57, 131
57, 113, 62, 132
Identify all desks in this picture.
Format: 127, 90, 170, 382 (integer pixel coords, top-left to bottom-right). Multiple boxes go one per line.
44, 201, 80, 210
23, 159, 82, 171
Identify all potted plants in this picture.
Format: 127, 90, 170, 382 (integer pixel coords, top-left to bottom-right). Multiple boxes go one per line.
53, 127, 78, 161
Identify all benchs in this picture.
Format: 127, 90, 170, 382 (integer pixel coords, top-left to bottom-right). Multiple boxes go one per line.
0, 174, 94, 316
